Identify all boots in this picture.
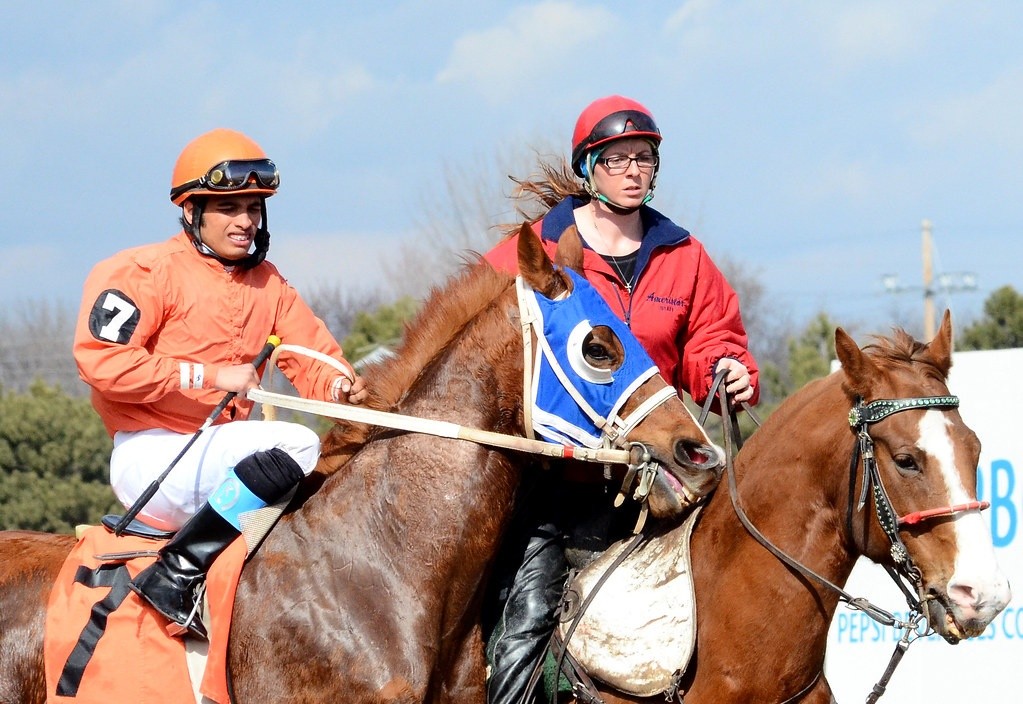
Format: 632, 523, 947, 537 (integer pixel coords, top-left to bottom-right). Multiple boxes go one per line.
127, 500, 242, 639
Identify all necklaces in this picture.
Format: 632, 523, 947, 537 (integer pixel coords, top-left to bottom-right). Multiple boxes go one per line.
592, 224, 635, 294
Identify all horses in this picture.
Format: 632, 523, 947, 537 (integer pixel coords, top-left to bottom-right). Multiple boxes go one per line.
0, 218, 1012, 704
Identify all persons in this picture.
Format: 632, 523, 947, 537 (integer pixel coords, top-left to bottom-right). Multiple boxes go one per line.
72, 128, 369, 643
478, 92, 761, 704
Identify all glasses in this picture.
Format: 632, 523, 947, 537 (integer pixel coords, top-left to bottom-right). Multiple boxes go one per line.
588, 110, 660, 145
597, 154, 659, 169
205, 158, 280, 190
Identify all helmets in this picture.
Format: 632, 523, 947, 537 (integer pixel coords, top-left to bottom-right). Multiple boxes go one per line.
570, 96, 664, 178
170, 129, 278, 206
